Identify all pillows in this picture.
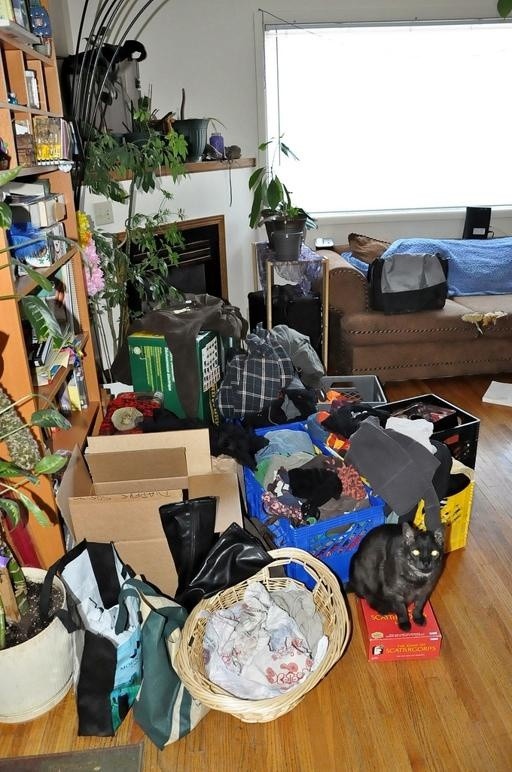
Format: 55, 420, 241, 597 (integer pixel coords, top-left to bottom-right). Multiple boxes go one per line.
348, 232, 391, 266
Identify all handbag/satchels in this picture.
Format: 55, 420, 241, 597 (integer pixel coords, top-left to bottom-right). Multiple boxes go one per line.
365, 250, 454, 319
31, 539, 212, 751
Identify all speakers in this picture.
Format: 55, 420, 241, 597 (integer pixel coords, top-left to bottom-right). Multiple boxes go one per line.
462, 207, 491, 239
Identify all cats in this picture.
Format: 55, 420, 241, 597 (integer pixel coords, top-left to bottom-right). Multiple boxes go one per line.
339, 520, 446, 631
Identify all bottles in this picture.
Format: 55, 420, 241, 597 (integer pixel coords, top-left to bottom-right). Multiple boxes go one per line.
210, 133, 224, 159
24, 69, 40, 109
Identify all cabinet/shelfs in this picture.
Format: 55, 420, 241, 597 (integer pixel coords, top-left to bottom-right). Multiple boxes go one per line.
0, 0, 104, 576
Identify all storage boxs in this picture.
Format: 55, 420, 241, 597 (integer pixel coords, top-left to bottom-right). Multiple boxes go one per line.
55, 428, 243, 599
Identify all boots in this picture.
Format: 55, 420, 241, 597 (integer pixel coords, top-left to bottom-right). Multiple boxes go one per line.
160, 496, 275, 615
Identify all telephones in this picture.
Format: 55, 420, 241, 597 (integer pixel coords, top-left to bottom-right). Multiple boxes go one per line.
315, 237, 334, 250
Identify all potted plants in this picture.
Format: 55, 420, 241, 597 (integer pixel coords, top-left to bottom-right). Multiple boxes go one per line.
247, 132, 318, 261
0, 136, 87, 724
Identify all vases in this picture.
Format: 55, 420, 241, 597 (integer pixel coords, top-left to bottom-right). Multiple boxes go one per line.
164, 119, 211, 163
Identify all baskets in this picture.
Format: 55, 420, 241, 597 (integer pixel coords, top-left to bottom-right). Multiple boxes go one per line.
169, 542, 354, 729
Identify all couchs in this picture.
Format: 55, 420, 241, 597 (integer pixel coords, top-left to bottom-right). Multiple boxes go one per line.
313, 235, 512, 384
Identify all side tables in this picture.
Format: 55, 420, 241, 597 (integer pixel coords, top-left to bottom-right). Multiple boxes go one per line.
251, 241, 329, 372
